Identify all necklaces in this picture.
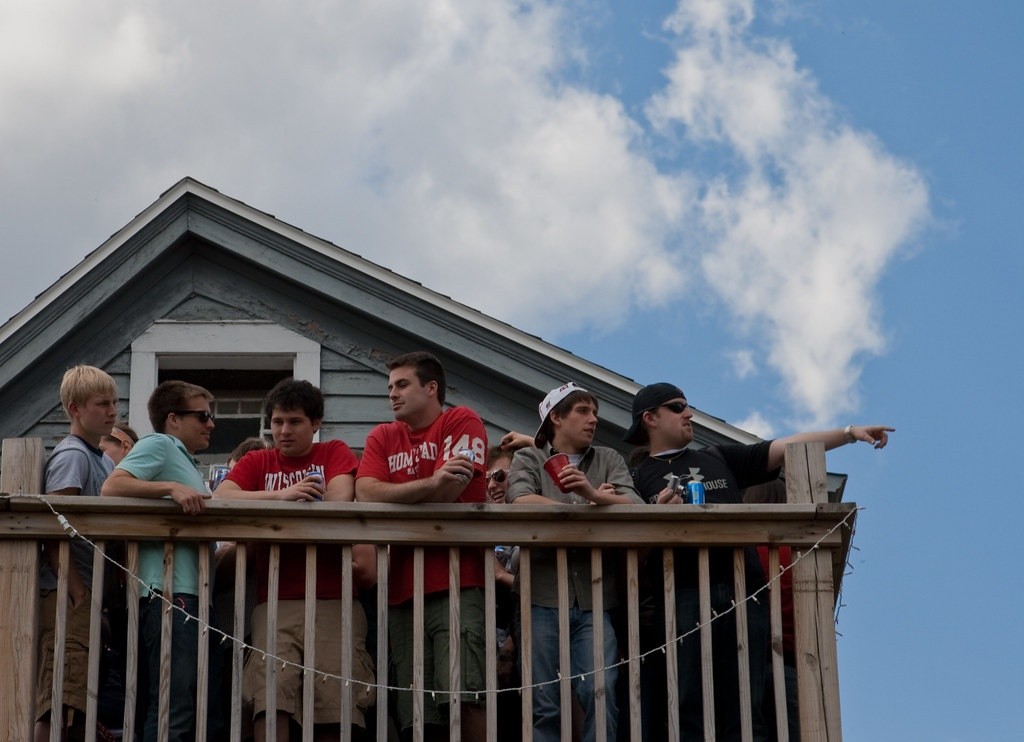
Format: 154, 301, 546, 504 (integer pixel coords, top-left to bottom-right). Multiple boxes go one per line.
649, 447, 688, 464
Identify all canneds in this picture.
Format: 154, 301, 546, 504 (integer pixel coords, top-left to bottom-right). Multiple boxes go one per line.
211, 466, 231, 491
455, 448, 475, 484
306, 472, 323, 502
495, 546, 509, 568
685, 480, 704, 504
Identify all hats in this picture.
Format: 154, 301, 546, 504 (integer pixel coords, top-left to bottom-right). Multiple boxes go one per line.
534, 381, 589, 450
623, 382, 687, 446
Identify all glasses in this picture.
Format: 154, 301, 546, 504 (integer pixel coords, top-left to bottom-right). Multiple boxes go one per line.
176, 410, 215, 425
657, 402, 687, 413
486, 469, 509, 484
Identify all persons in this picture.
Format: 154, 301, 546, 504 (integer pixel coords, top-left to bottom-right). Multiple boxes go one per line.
33, 349, 898, 742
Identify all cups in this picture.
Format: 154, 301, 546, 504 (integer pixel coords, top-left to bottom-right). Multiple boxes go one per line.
542, 452, 575, 494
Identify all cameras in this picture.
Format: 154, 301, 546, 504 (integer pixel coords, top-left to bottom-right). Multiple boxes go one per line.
669, 475, 685, 495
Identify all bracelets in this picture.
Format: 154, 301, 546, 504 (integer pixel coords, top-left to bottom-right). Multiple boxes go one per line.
844, 425, 857, 444
